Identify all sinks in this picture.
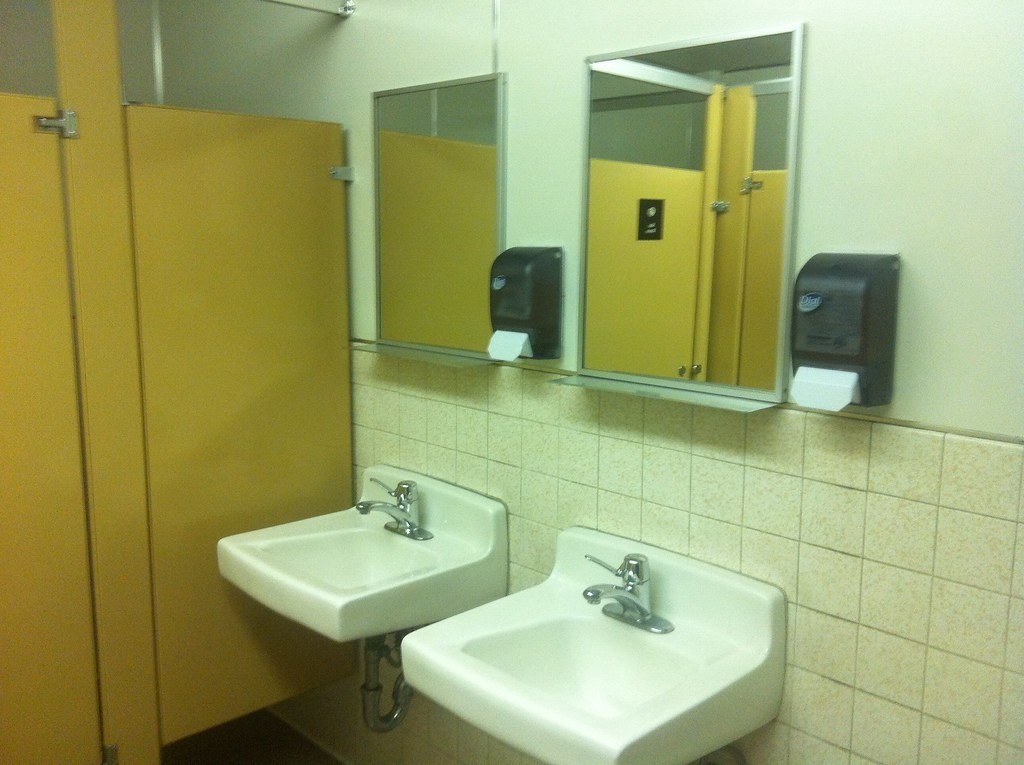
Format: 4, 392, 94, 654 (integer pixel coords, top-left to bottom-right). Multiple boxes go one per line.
214, 462, 508, 643
401, 524, 788, 765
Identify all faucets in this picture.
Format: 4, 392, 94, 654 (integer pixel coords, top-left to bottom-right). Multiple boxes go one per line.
355, 477, 433, 540
582, 553, 676, 634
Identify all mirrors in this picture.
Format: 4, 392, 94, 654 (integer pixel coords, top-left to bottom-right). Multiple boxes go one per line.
578, 21, 807, 406
372, 72, 508, 351
